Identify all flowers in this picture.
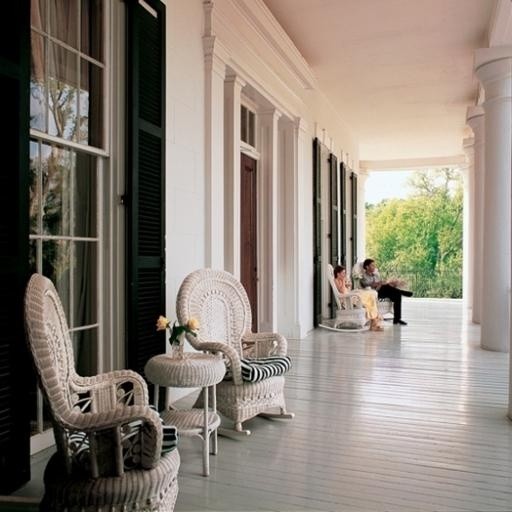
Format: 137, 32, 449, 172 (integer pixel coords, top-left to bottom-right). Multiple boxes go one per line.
155, 312, 203, 361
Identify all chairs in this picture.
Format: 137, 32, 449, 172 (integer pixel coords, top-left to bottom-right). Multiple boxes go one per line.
175, 266, 296, 439
351, 260, 397, 323
317, 262, 373, 334
22, 271, 183, 511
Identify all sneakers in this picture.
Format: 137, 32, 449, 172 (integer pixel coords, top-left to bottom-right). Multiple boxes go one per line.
368, 321, 383, 331
392, 292, 413, 325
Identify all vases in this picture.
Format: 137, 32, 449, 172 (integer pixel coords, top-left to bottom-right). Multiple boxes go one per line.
169, 342, 183, 362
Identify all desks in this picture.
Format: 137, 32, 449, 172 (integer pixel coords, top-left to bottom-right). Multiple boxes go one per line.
146, 347, 231, 479
349, 287, 378, 298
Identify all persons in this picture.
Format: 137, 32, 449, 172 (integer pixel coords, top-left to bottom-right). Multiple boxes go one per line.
333, 265, 384, 331
360, 259, 415, 325
